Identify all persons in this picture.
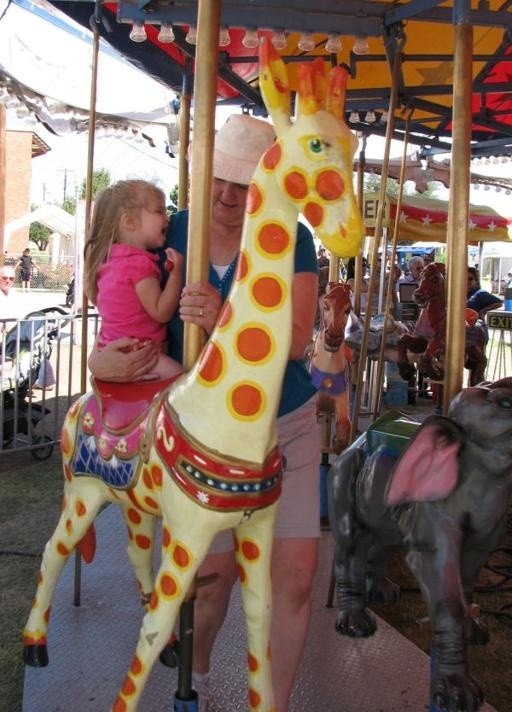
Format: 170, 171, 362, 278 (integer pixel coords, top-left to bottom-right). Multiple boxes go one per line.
88, 115, 321, 711
84, 179, 183, 381
15, 247, 40, 294
0, 266, 23, 333
315, 242, 512, 406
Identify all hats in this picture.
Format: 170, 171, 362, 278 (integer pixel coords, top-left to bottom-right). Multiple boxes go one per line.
318, 245, 325, 256
188, 114, 277, 186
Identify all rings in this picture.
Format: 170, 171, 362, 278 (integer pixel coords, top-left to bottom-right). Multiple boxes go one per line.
199, 307, 204, 317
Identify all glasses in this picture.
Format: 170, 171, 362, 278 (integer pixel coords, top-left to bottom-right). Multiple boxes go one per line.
417, 266, 423, 270
469, 277, 477, 281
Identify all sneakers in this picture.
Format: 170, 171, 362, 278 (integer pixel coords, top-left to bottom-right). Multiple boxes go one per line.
190, 672, 209, 712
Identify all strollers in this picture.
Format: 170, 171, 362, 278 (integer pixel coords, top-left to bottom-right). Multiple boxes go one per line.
0, 306, 70, 460
